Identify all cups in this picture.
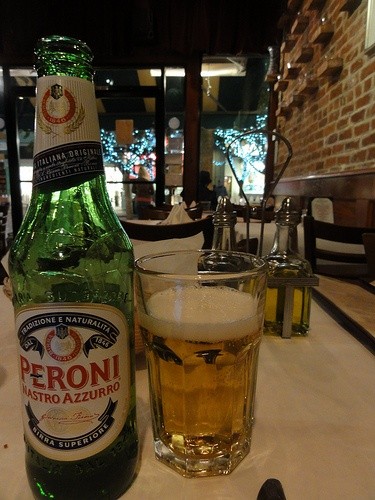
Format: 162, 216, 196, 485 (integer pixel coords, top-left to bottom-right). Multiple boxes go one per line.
134, 249, 268, 478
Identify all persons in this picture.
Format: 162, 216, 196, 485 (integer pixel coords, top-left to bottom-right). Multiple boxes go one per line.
132, 168, 155, 219
197, 172, 227, 211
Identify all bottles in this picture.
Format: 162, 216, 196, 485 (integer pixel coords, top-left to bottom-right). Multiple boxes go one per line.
9, 37, 138, 500
198, 196, 253, 273
264, 198, 313, 335
